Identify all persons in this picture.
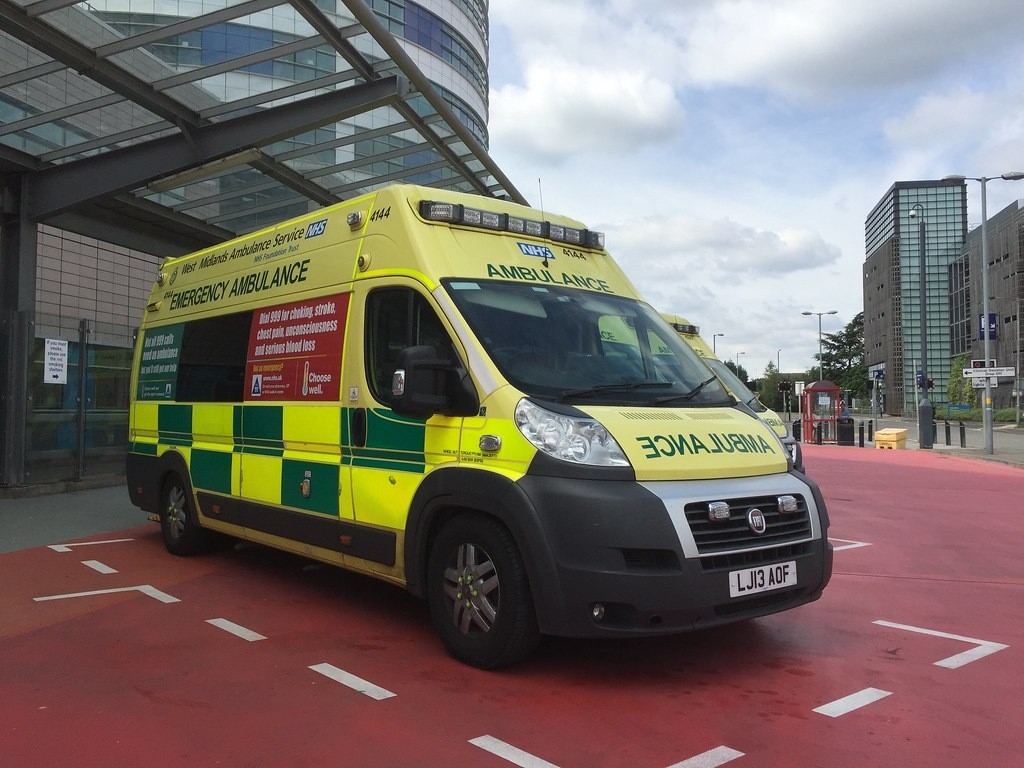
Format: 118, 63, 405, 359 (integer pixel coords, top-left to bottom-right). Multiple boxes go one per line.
840, 404, 850, 417
812, 408, 818, 438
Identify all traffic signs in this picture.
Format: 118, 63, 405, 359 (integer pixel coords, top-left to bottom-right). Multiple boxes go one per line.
962, 366, 1016, 378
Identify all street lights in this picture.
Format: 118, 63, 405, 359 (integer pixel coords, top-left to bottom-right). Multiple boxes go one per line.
713, 334, 724, 354
777, 349, 781, 373
736, 352, 745, 377
909, 203, 935, 451
941, 171, 1024, 455
802, 310, 838, 432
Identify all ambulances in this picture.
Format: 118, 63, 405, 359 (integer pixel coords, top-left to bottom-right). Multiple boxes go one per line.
123, 186, 839, 674
600, 317, 806, 475
28, 362, 134, 469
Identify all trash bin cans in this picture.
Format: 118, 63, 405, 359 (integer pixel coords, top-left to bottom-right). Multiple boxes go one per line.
792, 420, 802, 441
837, 417, 854, 446
